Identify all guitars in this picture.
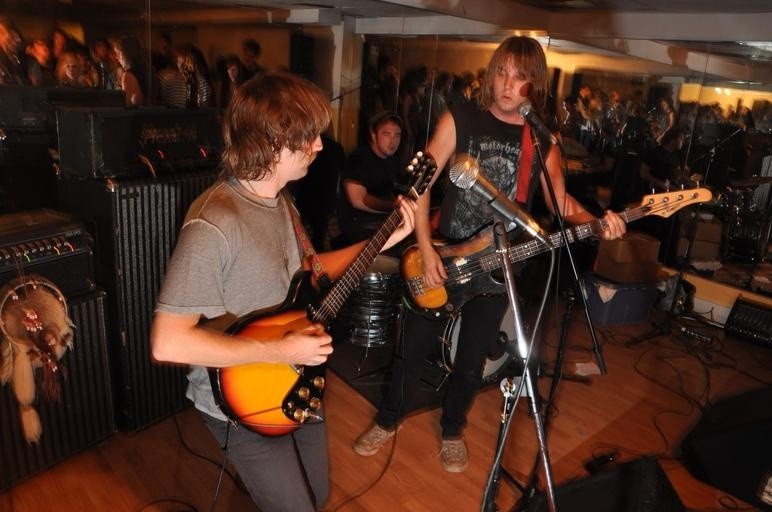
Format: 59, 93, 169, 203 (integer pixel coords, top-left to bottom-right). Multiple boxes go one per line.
208, 152, 438, 439
399, 174, 712, 319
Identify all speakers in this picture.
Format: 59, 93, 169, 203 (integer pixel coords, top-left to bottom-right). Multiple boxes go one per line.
504, 456, 690, 512
674, 388, 772, 512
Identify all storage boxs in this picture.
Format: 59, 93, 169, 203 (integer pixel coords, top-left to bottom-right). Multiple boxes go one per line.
676, 208, 722, 260
571, 231, 661, 326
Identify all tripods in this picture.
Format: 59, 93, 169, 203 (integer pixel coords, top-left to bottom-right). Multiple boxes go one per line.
623, 125, 740, 349
502, 164, 589, 402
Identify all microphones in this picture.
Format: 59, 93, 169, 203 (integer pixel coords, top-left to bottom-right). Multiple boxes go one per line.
517, 101, 562, 148
446, 159, 551, 249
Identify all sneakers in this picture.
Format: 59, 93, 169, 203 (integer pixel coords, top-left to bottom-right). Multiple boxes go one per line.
437, 434, 469, 474
353, 414, 402, 457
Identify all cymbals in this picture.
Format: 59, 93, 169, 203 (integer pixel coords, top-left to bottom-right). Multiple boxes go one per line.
732, 176, 771, 188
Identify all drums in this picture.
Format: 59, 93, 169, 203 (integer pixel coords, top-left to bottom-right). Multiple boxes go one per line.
342, 255, 400, 348
402, 278, 531, 393
